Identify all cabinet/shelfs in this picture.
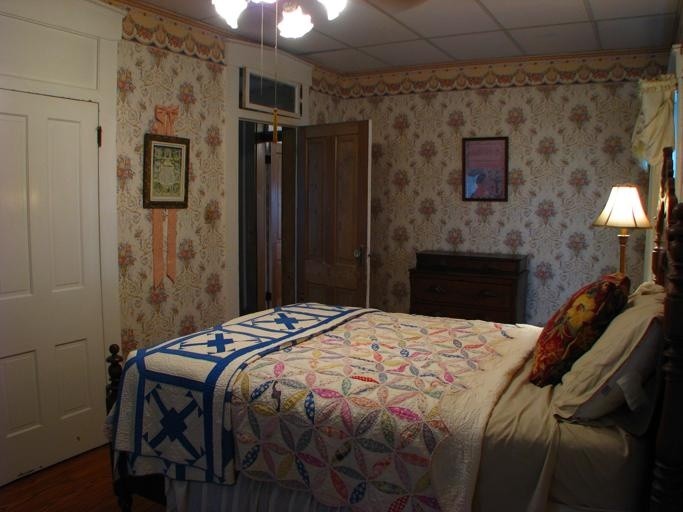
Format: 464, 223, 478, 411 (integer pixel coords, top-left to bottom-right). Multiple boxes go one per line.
407, 249, 531, 324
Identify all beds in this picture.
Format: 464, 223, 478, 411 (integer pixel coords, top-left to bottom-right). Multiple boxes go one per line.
104, 147, 682, 512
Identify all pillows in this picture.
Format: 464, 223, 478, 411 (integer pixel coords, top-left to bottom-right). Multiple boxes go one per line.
528, 274, 666, 423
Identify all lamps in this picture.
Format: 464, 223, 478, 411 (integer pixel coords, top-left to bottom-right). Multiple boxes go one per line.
593, 184, 648, 275
211, 0, 350, 39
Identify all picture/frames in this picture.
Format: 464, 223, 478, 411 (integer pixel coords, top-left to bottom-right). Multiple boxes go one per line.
461, 136, 508, 202
144, 133, 190, 209
